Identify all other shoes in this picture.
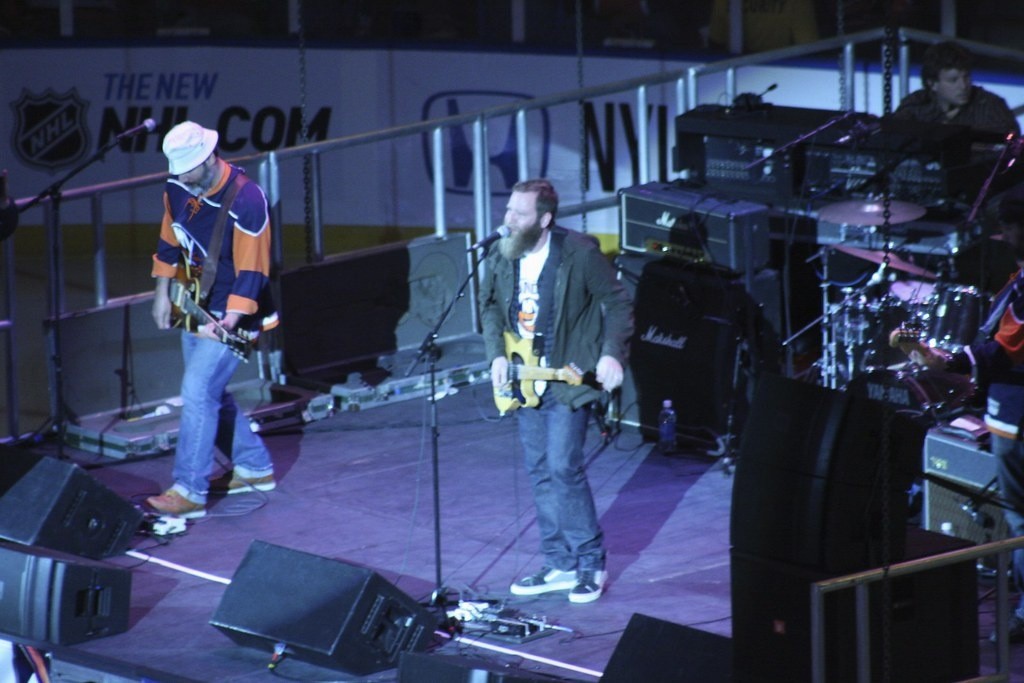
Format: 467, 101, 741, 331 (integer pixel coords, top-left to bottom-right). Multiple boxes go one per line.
989, 617, 1023, 642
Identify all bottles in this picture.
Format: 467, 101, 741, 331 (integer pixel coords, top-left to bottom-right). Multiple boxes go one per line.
659, 399, 677, 454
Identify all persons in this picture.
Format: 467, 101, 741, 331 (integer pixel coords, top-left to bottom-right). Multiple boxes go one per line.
908, 187, 1024, 644
146, 121, 280, 518
891, 41, 1020, 134
477, 179, 635, 602
708, 0, 817, 55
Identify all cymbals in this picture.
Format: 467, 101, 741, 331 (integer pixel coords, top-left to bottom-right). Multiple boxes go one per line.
820, 242, 940, 281
817, 197, 931, 227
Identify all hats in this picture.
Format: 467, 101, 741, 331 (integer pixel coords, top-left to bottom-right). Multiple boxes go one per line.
162, 121, 218, 175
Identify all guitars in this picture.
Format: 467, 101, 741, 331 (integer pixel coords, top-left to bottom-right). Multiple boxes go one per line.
491, 329, 604, 418
166, 256, 254, 360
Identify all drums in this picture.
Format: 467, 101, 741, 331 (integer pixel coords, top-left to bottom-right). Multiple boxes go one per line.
809, 286, 999, 417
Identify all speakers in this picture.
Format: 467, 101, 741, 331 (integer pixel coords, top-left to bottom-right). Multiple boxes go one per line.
927, 476, 1020, 571
0, 445, 143, 649
395, 253, 926, 683
207, 539, 438, 677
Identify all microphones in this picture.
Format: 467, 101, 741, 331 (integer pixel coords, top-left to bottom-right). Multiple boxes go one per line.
466, 225, 512, 252
960, 501, 993, 529
836, 123, 883, 145
117, 120, 156, 139
1009, 136, 1024, 169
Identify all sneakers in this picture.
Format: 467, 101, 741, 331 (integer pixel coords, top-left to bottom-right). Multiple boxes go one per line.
210, 472, 276, 495
145, 488, 208, 519
568, 568, 609, 603
509, 565, 579, 595
